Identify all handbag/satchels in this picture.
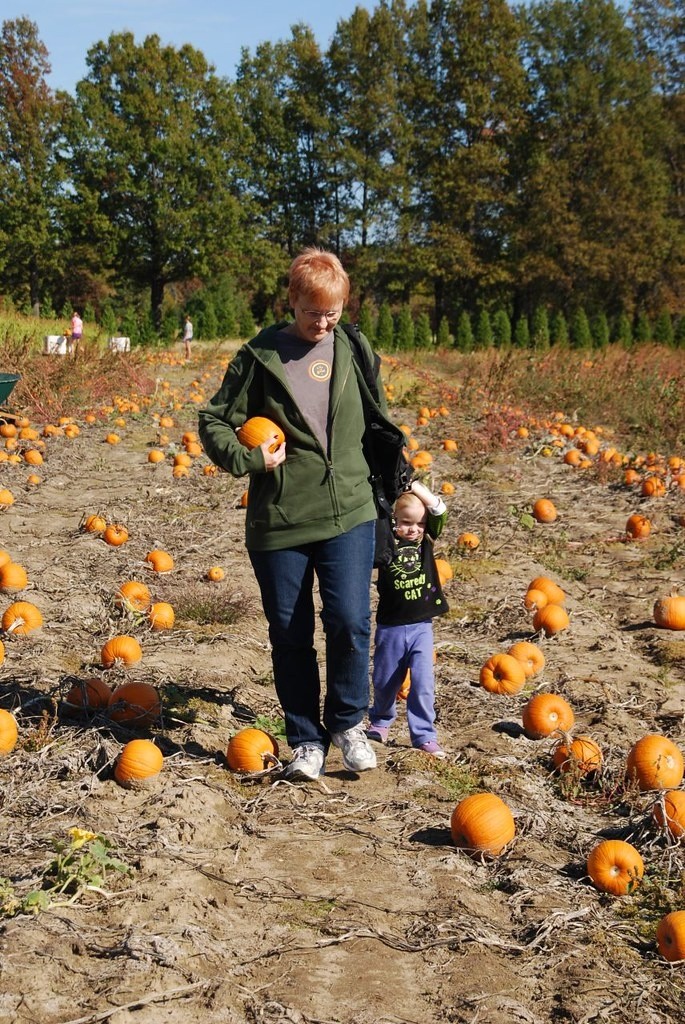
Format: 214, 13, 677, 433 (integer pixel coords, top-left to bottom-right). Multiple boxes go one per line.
361, 416, 415, 569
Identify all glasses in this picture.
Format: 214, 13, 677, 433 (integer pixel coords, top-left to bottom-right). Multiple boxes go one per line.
293, 294, 339, 320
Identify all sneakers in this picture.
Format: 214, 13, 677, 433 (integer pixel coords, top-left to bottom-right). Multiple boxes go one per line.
366, 723, 390, 743
283, 743, 326, 781
418, 738, 445, 760
330, 721, 378, 773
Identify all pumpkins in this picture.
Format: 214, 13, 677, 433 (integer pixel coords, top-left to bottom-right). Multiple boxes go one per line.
382, 380, 685, 856
0, 351, 286, 792
587, 840, 645, 894
654, 909, 685, 962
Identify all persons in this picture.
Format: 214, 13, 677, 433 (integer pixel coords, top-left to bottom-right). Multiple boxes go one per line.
68, 311, 83, 354
366, 480, 448, 756
199, 250, 412, 780
184, 316, 193, 362
